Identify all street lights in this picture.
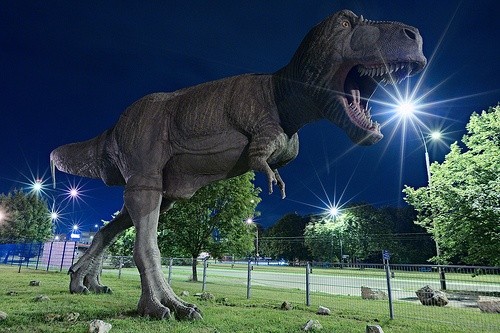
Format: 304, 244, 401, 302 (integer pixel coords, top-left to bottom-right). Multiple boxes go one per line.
330, 207, 343, 261
247, 219, 258, 258
18, 182, 41, 273
396, 102, 442, 275
